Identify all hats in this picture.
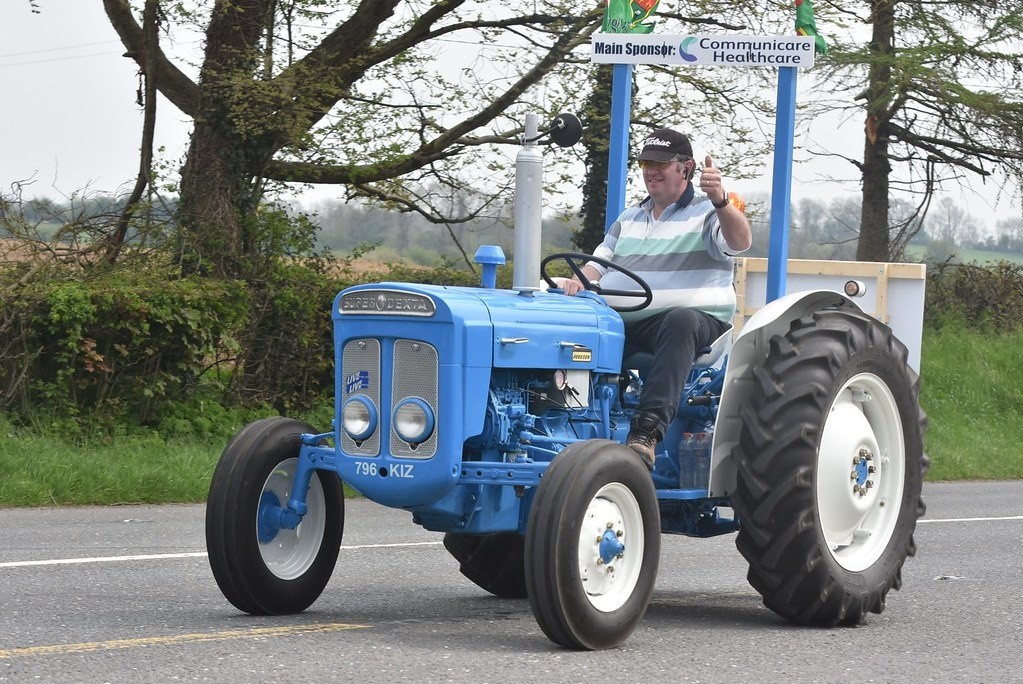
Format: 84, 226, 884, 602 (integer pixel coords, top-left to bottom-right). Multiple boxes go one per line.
638, 128, 693, 162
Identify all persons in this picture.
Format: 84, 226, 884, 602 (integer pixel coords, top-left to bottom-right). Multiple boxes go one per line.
524, 128, 752, 470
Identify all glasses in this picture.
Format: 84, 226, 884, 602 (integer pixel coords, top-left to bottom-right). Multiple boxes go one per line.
638, 159, 684, 170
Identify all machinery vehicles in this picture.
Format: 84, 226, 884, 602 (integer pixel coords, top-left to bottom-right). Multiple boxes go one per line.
202, 3, 935, 650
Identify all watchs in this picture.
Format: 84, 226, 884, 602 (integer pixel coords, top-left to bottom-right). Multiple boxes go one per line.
711, 192, 730, 208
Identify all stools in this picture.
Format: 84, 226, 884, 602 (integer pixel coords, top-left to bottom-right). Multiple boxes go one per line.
626, 322, 735, 369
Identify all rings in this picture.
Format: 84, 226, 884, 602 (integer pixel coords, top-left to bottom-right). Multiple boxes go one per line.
707, 180, 710, 186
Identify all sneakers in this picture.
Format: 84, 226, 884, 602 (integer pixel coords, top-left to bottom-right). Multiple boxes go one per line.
625, 412, 659, 471
